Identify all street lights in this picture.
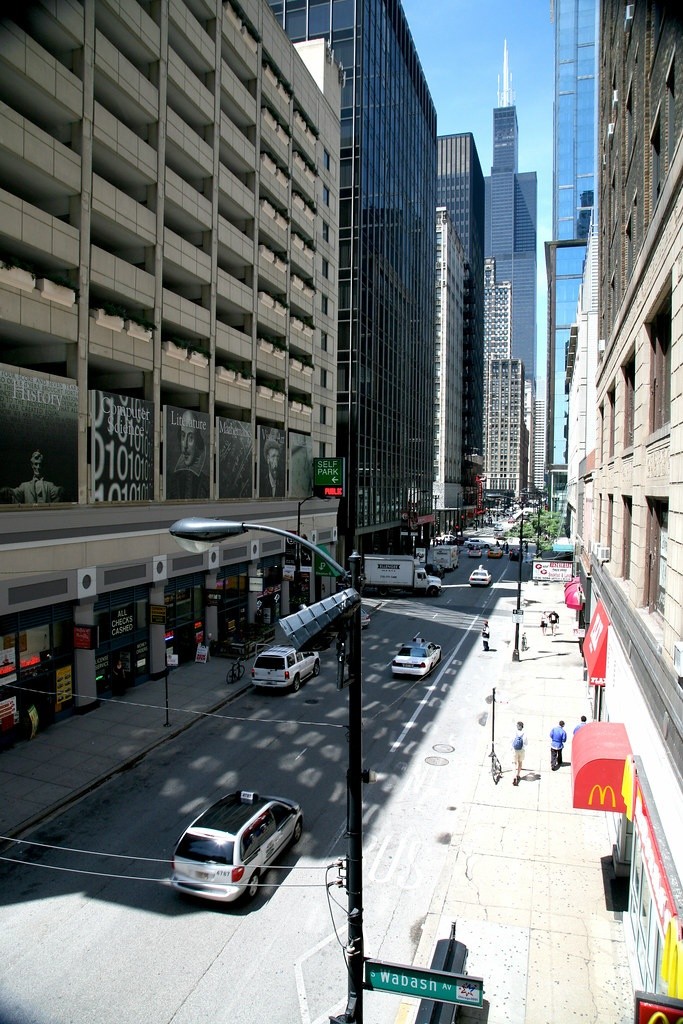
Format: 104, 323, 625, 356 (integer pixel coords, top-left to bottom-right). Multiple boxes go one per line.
408, 490, 429, 553
457, 490, 470, 539
169, 514, 364, 1023
524, 491, 540, 556
487, 493, 523, 662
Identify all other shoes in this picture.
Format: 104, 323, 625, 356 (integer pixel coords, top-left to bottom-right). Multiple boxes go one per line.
514, 776, 520, 785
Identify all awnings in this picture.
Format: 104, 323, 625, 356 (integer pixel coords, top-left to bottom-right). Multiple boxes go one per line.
563, 576, 584, 611
582, 599, 612, 687
571, 722, 635, 813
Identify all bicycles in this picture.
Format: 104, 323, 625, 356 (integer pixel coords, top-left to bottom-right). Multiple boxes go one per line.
225, 656, 245, 683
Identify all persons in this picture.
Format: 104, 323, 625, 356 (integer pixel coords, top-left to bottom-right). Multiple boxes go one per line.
573, 716, 587, 735
520, 632, 530, 651
433, 538, 445, 548
481, 619, 490, 651
547, 611, 560, 637
510, 721, 528, 786
501, 541, 509, 554
495, 540, 500, 549
540, 611, 549, 636
549, 721, 567, 771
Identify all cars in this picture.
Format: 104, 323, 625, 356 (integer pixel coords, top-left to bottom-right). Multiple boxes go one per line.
173, 790, 305, 908
440, 516, 515, 549
467, 544, 482, 558
251, 643, 320, 692
468, 564, 491, 586
390, 637, 442, 679
424, 563, 445, 579
509, 548, 523, 561
361, 608, 370, 630
488, 546, 504, 558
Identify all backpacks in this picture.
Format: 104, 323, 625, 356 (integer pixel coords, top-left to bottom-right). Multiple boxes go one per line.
514, 731, 523, 750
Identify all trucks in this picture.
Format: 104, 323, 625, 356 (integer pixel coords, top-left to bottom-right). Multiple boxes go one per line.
433, 545, 459, 571
361, 553, 442, 596
